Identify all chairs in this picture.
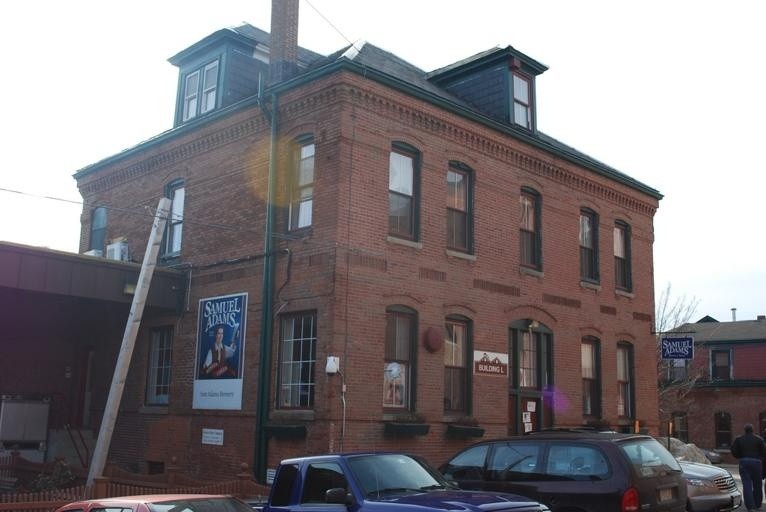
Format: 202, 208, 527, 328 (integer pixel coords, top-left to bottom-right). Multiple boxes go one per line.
570, 457, 591, 474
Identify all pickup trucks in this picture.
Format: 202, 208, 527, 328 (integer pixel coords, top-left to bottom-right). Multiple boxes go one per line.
248, 454, 551, 512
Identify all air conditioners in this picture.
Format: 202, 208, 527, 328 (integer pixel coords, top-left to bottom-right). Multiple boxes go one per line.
107, 242, 128, 261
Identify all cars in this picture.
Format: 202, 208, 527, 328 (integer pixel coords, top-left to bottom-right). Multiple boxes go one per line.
54, 494, 256, 512
673, 456, 743, 512
655, 436, 722, 465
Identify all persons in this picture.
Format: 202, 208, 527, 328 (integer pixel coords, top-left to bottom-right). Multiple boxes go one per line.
200, 324, 237, 379
730, 422, 765, 512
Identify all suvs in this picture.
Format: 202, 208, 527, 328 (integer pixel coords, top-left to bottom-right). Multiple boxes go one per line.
438, 424, 688, 512
